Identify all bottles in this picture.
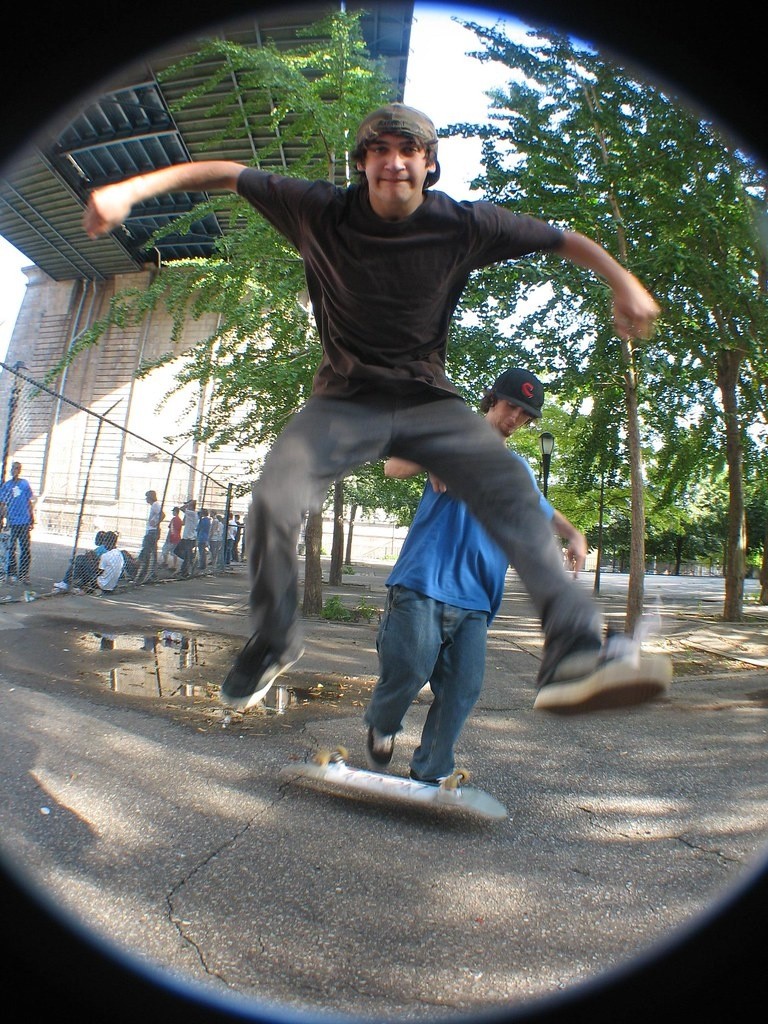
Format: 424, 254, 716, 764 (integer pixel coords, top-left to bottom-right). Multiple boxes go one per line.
157, 630, 182, 643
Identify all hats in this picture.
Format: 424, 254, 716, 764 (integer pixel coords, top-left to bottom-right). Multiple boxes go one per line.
171, 507, 179, 512
492, 368, 544, 418
357, 102, 441, 188
184, 499, 195, 506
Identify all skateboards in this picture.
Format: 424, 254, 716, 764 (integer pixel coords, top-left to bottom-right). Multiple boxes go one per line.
275, 742, 513, 824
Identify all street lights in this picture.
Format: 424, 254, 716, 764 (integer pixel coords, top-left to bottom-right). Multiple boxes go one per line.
538, 432, 556, 500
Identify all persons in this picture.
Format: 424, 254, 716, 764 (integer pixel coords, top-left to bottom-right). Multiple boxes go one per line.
0, 462, 35, 586
162, 500, 245, 577
53, 531, 124, 593
363, 368, 587, 790
120, 490, 165, 584
93, 632, 118, 650
85, 104, 674, 711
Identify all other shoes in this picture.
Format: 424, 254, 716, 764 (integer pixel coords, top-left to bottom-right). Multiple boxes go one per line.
19, 572, 31, 585
70, 588, 81, 595
158, 563, 168, 569
369, 729, 397, 767
166, 566, 177, 572
53, 581, 68, 590
531, 630, 674, 715
221, 628, 305, 708
5, 575, 19, 586
408, 768, 461, 790
